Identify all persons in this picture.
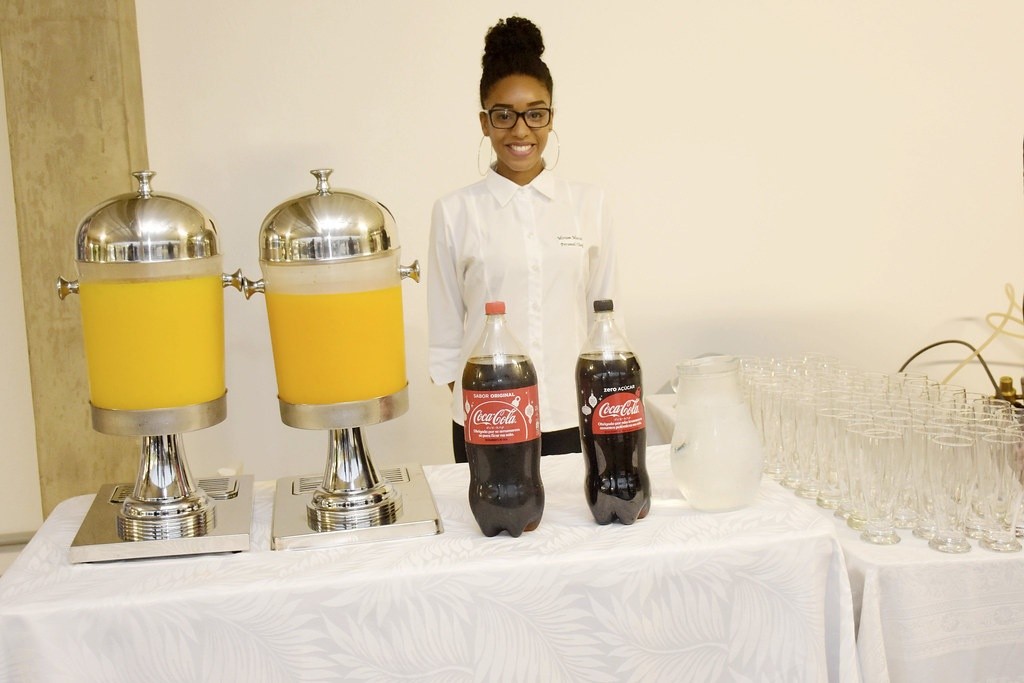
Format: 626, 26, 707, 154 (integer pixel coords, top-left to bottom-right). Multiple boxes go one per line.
427, 16, 618, 463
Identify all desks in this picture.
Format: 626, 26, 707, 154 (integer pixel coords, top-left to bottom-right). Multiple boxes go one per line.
0, 438, 862, 682
645, 391, 1024, 682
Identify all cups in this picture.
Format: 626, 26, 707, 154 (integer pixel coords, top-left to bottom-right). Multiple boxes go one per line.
670, 353, 1024, 553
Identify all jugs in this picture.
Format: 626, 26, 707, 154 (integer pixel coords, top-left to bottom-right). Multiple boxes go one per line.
670, 355, 761, 512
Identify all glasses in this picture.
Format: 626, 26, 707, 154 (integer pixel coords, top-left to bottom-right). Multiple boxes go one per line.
479, 105, 555, 129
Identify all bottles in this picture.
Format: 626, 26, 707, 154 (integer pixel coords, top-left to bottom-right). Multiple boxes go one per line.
461, 303, 546, 538
576, 301, 651, 527
